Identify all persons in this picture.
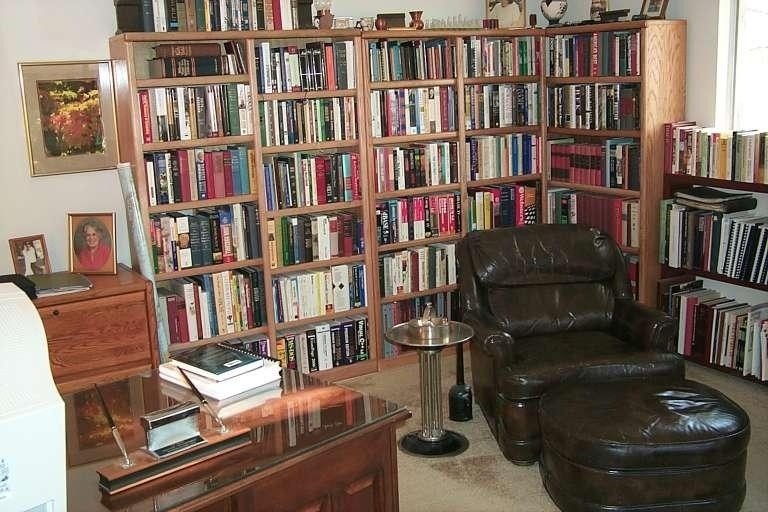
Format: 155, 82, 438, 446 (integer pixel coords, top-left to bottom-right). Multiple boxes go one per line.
78, 220, 111, 270
21, 241, 37, 277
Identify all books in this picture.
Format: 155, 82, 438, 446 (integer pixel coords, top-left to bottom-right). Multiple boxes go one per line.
461, 33, 539, 227
656, 121, 767, 382
369, 38, 467, 363
159, 342, 282, 420
255, 38, 374, 372
116, 0, 317, 31
545, 30, 642, 302
129, 31, 271, 376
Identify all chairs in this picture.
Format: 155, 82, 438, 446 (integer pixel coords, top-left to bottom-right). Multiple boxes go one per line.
454, 222, 687, 467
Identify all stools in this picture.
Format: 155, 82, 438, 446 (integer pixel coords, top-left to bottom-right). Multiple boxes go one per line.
539, 374, 750, 512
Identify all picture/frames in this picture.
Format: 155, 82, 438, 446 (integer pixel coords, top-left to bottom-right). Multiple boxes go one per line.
8, 234, 52, 278
17, 58, 121, 177
640, 0, 669, 19
485, 0, 527, 30
68, 211, 118, 276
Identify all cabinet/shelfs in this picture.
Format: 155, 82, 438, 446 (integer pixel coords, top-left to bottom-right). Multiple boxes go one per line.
362, 27, 546, 371
108, 26, 379, 405
543, 19, 687, 310
663, 173, 768, 388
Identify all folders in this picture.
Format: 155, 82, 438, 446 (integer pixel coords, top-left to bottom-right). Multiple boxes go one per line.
25, 271, 92, 300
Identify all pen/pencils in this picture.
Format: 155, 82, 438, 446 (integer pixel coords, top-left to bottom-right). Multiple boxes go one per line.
93, 382, 130, 465
176, 366, 226, 432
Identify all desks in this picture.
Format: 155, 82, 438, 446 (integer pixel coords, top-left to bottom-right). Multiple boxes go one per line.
59, 360, 414, 512
29, 262, 163, 468
383, 319, 474, 459
